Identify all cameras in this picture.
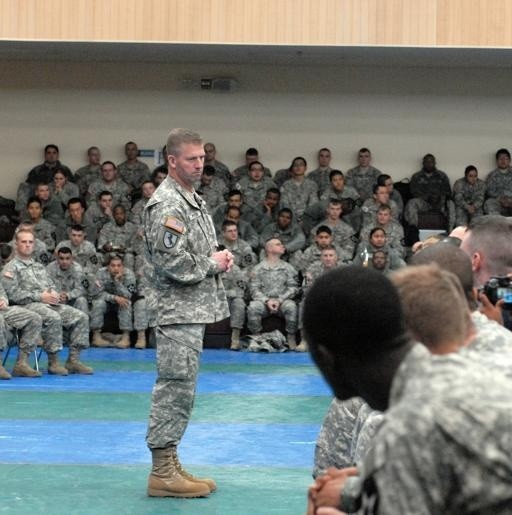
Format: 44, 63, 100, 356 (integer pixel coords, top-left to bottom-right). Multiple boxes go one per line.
477, 273, 512, 309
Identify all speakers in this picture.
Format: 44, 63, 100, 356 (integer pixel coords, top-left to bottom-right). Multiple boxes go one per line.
212, 79, 239, 93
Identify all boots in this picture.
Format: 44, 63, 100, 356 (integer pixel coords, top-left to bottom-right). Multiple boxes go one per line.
228, 326, 313, 354
91, 328, 148, 350
46, 351, 68, 378
147, 445, 218, 499
0, 358, 12, 381
12, 349, 42, 378
65, 350, 93, 376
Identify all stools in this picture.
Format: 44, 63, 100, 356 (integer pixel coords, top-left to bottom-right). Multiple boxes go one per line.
418, 209, 445, 229
2, 324, 52, 374
